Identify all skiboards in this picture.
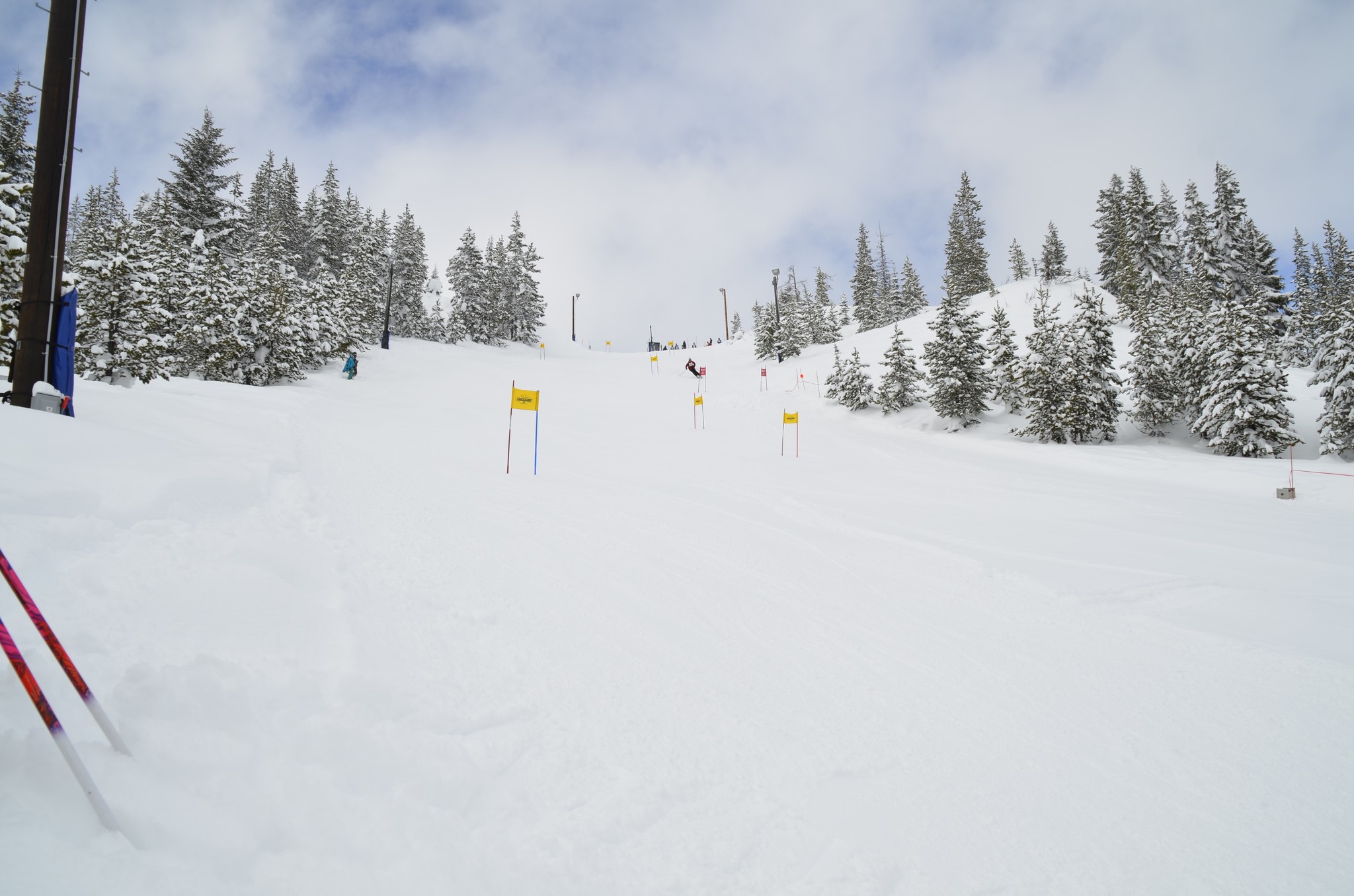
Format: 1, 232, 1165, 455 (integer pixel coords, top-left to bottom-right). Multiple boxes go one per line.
697, 376, 702, 379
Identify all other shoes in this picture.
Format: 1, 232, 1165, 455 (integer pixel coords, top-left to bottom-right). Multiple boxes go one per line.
700, 376, 702, 378
697, 376, 699, 378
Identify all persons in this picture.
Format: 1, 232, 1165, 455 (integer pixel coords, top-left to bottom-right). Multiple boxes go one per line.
352, 352, 358, 377
342, 355, 355, 380
683, 341, 686, 349
682, 345, 684, 349
670, 345, 675, 350
685, 358, 702, 379
717, 337, 721, 343
692, 342, 697, 348
706, 341, 710, 346
675, 343, 679, 349
663, 346, 668, 351
709, 338, 712, 345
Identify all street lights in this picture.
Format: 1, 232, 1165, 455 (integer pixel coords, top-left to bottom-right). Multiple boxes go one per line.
719, 288, 729, 340
380, 251, 396, 349
771, 268, 783, 363
572, 293, 580, 342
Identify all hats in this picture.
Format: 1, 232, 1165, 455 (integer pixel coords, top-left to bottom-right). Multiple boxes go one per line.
353, 352, 357, 357
688, 358, 691, 360
